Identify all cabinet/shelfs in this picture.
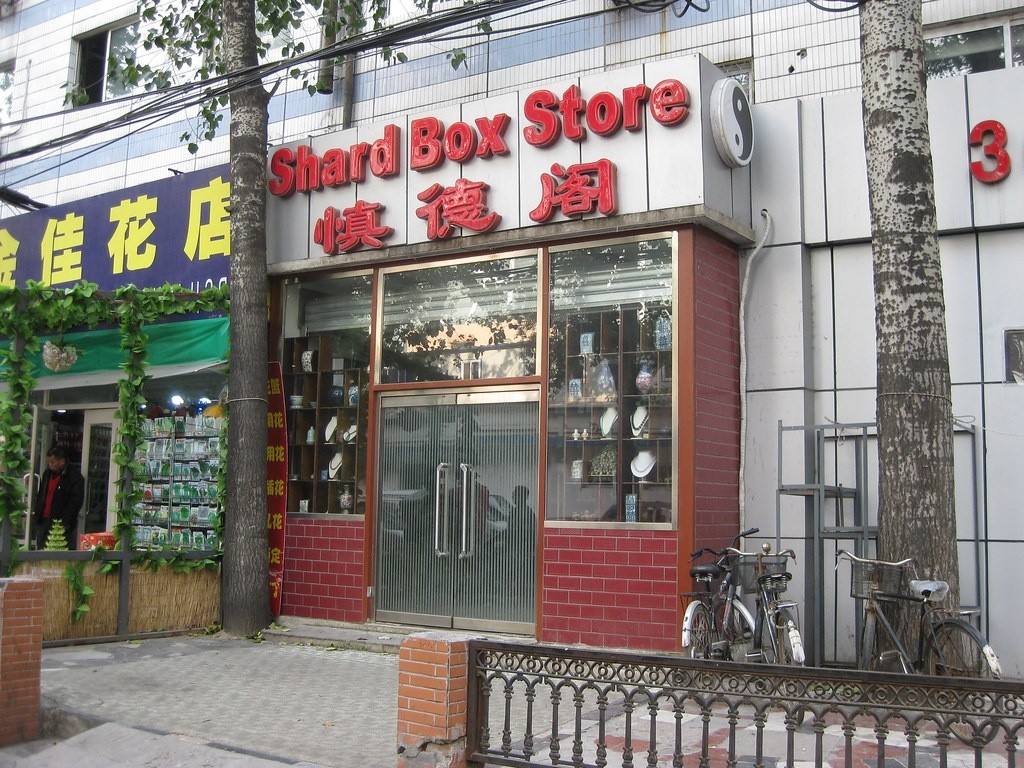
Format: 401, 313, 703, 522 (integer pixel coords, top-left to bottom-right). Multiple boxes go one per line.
556, 302, 672, 523
129, 426, 224, 552
288, 336, 370, 515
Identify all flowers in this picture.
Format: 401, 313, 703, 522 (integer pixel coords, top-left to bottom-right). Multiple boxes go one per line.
43, 340, 78, 373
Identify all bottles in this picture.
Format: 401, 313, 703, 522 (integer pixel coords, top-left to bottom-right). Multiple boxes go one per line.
635, 360, 653, 394
339, 485, 353, 514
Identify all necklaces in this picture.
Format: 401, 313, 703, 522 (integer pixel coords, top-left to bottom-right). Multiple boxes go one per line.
345, 428, 357, 441
634, 456, 656, 473
330, 458, 342, 470
601, 412, 618, 436
631, 410, 648, 431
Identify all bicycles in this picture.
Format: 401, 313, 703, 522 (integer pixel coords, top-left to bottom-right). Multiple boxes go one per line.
679, 528, 760, 688
725, 542, 807, 725
835, 549, 1002, 747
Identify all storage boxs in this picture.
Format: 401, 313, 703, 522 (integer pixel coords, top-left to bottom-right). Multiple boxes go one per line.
80, 531, 118, 550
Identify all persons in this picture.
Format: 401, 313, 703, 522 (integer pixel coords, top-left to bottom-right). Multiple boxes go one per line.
399, 461, 535, 553
31, 447, 85, 550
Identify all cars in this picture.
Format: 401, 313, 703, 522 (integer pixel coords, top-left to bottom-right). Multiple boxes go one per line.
346, 488, 520, 594
